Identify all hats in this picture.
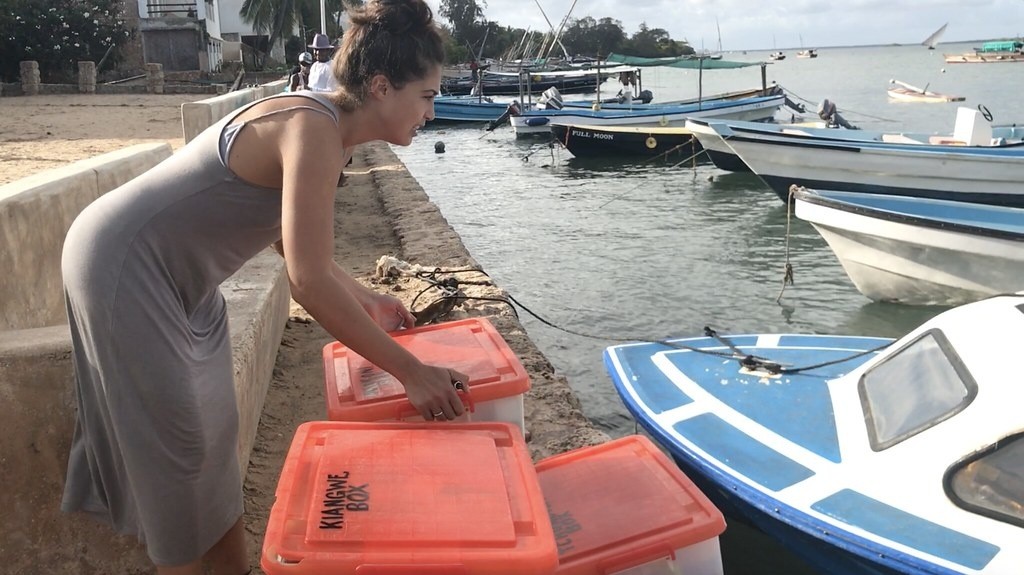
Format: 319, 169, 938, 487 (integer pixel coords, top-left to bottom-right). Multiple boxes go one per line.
307, 34, 335, 50
299, 52, 312, 65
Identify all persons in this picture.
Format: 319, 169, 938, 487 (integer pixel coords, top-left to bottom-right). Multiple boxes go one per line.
296, 34, 347, 187
59, 0, 470, 575
288, 52, 312, 92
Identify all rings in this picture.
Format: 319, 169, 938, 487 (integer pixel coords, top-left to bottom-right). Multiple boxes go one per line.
434, 412, 442, 416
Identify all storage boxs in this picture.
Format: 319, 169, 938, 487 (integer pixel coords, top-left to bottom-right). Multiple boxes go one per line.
321, 317, 530, 417
262, 421, 561, 574
531, 436, 727, 575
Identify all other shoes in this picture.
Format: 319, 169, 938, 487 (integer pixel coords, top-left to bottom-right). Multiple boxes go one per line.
337, 172, 347, 187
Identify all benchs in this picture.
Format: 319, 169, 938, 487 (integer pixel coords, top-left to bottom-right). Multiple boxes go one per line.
0, 79, 292, 575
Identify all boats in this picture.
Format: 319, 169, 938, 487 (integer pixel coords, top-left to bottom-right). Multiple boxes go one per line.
886, 77, 967, 103
433, 0, 807, 159
680, 96, 1024, 174
941, 39, 1024, 64
708, 101, 1024, 210
600, 290, 1024, 574
791, 184, 1024, 309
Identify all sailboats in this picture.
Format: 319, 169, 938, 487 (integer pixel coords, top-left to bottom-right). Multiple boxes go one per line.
795, 34, 818, 58
921, 21, 950, 50
767, 31, 787, 61
